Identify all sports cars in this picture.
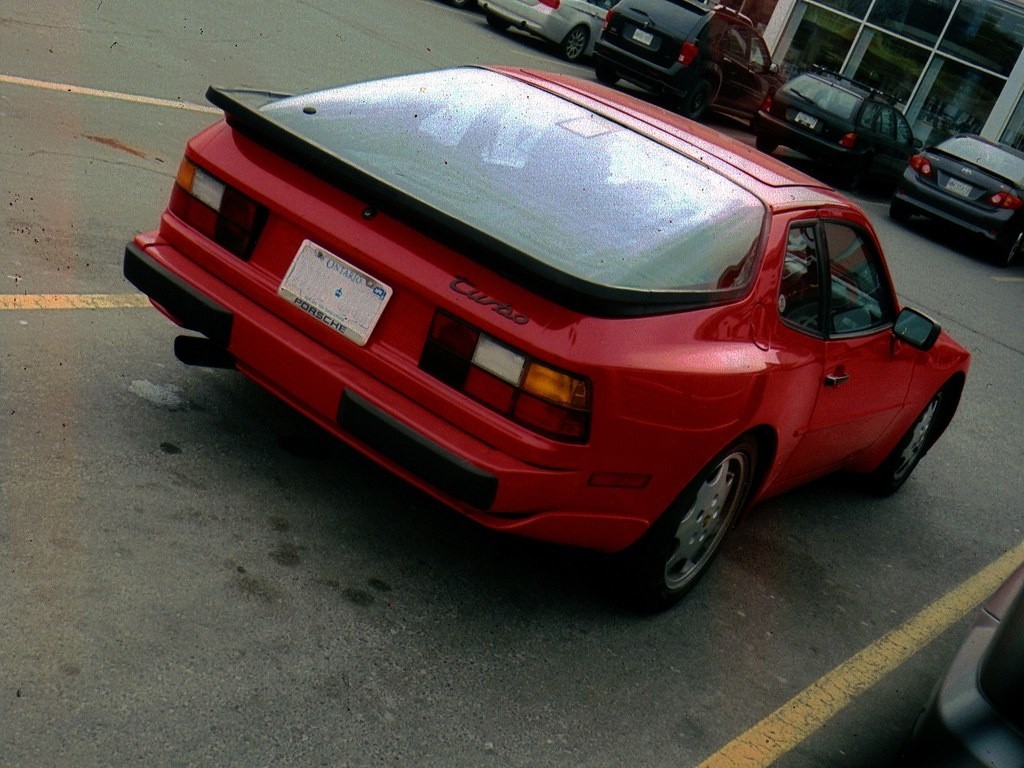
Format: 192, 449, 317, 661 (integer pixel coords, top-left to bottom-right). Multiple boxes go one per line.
123, 67, 970, 618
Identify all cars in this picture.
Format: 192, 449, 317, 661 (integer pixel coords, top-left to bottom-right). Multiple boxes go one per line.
889, 132, 1024, 269
755, 65, 924, 193
477, 0, 620, 63
904, 560, 1024, 768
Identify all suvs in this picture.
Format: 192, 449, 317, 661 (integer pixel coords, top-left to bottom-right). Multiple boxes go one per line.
592, 0, 787, 126
